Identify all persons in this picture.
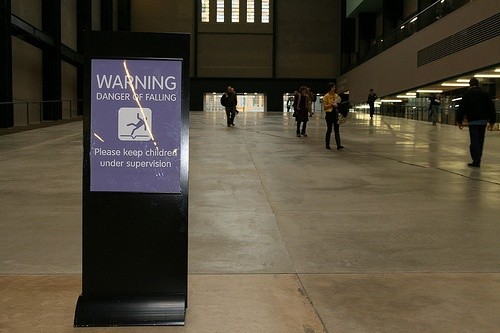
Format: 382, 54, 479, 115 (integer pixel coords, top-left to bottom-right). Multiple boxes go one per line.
428, 94, 440, 125
220, 87, 240, 128
293, 86, 315, 137
323, 82, 345, 149
367, 89, 378, 118
457, 78, 496, 167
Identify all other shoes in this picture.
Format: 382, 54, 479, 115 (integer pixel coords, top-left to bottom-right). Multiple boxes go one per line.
337, 146, 344, 150
468, 163, 479, 167
303, 134, 307, 136
326, 146, 331, 149
296, 133, 302, 137
